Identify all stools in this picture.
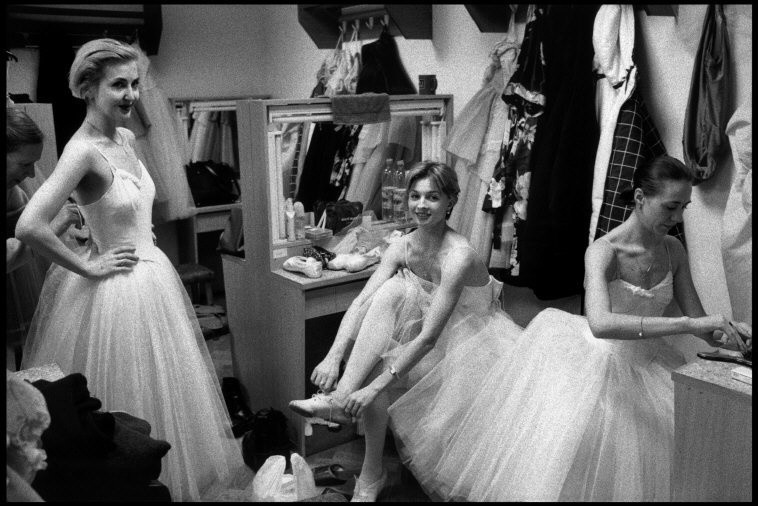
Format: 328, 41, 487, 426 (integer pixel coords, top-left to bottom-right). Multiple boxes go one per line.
176, 263, 215, 307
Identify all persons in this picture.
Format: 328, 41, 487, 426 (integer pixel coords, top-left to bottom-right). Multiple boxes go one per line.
287, 160, 493, 502
8, 103, 81, 274
16, 36, 253, 501
436, 155, 751, 501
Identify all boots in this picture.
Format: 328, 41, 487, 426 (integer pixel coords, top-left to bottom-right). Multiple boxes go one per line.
221, 377, 258, 439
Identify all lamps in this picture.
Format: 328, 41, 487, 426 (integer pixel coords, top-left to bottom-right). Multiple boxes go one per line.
268, 99, 445, 125
186, 101, 237, 113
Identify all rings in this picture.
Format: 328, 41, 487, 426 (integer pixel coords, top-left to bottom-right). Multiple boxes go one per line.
320, 381, 324, 385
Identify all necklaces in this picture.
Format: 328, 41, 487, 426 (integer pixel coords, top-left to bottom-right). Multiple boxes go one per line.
624, 222, 657, 281
416, 226, 444, 279
84, 117, 128, 157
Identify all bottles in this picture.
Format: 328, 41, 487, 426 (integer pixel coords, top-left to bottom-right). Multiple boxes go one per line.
392, 159, 410, 225
381, 158, 396, 222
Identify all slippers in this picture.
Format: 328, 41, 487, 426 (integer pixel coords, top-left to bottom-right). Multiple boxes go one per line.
311, 464, 362, 486
322, 476, 357, 501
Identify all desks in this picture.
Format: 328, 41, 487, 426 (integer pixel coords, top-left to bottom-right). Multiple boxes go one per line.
671, 347, 753, 503
177, 203, 241, 306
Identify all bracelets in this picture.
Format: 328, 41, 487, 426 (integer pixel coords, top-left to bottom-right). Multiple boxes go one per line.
388, 363, 400, 379
639, 314, 647, 339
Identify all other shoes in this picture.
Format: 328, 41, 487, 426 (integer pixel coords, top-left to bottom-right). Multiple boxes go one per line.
327, 246, 382, 273
282, 255, 323, 278
350, 467, 388, 502
288, 390, 352, 428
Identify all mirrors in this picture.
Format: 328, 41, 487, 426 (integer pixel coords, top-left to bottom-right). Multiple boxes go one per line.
174, 99, 241, 208
270, 99, 448, 248
